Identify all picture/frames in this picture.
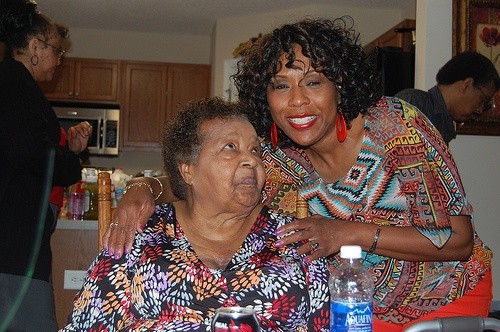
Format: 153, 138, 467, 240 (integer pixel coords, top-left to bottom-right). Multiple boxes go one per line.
452, 0, 500, 137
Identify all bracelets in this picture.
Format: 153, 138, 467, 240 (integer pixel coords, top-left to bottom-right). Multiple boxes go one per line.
145, 176, 164, 200
122, 182, 153, 194
369, 225, 384, 253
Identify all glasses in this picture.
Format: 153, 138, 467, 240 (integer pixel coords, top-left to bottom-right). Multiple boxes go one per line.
478, 87, 491, 107
38, 37, 65, 59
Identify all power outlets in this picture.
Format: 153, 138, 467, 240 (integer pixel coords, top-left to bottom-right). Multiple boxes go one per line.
64, 270, 87, 290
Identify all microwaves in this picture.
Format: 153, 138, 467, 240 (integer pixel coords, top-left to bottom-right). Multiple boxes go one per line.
48, 100, 120, 156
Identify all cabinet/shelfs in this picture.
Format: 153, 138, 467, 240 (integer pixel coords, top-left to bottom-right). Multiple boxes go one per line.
360, 19, 417, 62
35, 56, 212, 152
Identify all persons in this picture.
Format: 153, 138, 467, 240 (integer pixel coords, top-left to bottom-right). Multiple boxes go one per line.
59, 96, 329, 332
389, 50, 500, 148
0, 0, 92, 332
100, 20, 492, 332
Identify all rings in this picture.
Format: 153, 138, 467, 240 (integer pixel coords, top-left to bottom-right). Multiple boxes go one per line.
109, 221, 120, 229
311, 243, 318, 249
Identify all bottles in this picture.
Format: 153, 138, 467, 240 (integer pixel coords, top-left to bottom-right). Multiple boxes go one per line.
67, 166, 154, 221
328, 244, 376, 332
